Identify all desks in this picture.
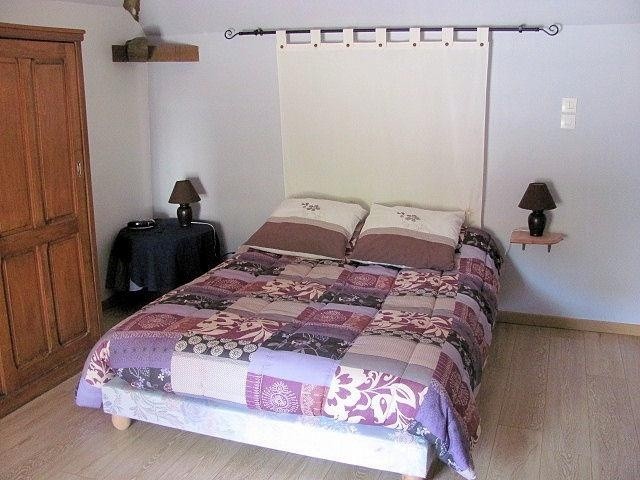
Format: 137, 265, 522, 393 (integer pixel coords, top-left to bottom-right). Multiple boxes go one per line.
106, 219, 221, 294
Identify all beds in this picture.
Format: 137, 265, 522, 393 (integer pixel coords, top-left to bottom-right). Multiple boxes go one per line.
75, 228, 505, 480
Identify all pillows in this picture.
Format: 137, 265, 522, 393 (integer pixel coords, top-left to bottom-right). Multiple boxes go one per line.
347, 204, 466, 271
242, 197, 367, 263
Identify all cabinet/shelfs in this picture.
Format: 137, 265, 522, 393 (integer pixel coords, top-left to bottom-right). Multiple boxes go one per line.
1, 22, 106, 418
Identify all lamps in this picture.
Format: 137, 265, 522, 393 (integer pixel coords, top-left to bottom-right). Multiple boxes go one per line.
170, 180, 202, 226
518, 183, 557, 236
